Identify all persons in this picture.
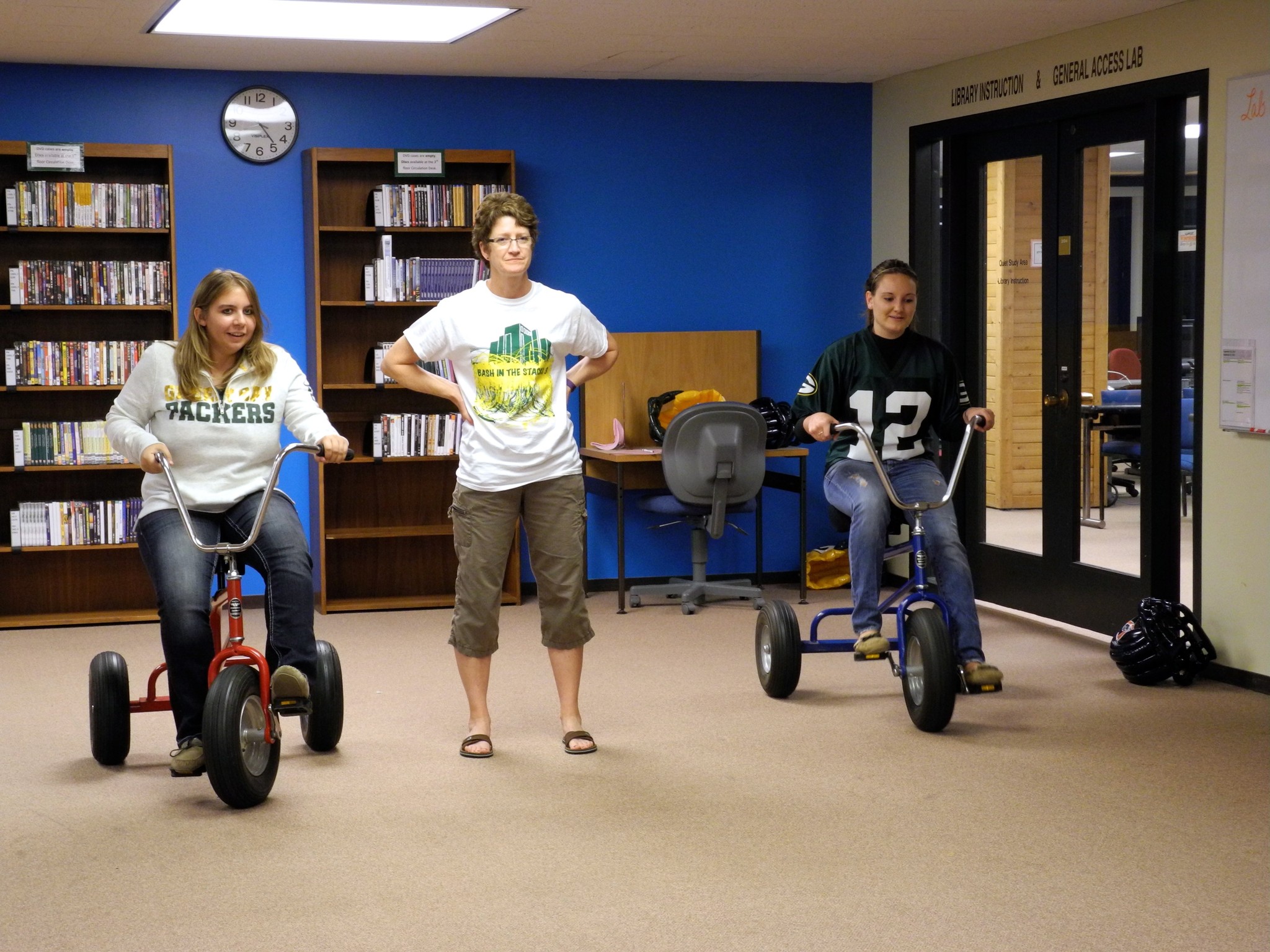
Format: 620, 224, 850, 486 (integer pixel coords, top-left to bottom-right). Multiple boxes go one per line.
790, 259, 1003, 685
380, 192, 620, 758
103, 269, 350, 775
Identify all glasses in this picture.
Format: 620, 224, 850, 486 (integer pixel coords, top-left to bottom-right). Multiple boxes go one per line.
485, 236, 533, 245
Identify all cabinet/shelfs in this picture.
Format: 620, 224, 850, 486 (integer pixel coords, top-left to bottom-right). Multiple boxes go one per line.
301, 147, 521, 615
0, 141, 180, 627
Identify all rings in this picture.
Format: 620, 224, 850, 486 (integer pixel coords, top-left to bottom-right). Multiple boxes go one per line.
818, 431, 823, 435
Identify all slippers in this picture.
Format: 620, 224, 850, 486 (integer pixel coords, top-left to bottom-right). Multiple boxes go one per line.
459, 734, 493, 758
562, 730, 597, 754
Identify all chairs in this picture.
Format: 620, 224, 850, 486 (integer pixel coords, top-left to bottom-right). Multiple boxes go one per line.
1099, 348, 1193, 516
629, 402, 766, 615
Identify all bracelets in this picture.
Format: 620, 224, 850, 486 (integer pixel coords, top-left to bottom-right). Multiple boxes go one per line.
566, 378, 576, 392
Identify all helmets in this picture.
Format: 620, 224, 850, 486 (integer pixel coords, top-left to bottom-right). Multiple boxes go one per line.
1110, 596, 1216, 688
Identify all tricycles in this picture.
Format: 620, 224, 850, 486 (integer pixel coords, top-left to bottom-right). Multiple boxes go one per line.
753, 414, 1002, 734
87, 442, 355, 810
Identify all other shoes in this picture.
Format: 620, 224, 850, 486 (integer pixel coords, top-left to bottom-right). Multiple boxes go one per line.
964, 664, 1003, 684
169, 734, 204, 774
270, 665, 310, 703
854, 632, 890, 654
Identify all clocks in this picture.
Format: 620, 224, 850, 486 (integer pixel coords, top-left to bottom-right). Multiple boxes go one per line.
220, 85, 299, 165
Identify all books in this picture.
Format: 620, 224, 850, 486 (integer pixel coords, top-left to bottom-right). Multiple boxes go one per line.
373, 412, 464, 458
374, 184, 511, 227
10, 497, 145, 547
364, 235, 491, 301
5, 180, 170, 228
5, 340, 158, 385
9, 260, 171, 305
13, 421, 129, 466
374, 342, 455, 384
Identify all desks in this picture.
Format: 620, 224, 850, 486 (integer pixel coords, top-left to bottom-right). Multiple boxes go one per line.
579, 447, 809, 614
